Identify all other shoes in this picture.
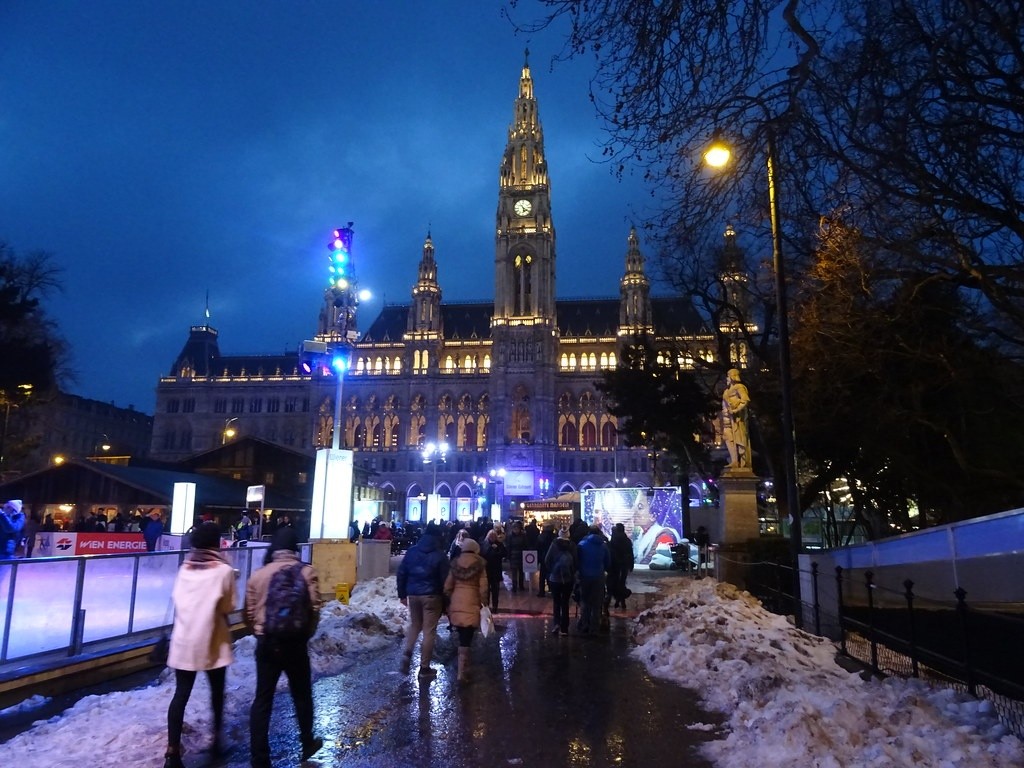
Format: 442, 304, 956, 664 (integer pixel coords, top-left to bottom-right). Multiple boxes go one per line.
301, 738, 324, 761
511, 588, 517, 593
552, 625, 560, 633
560, 631, 567, 635
399, 655, 410, 673
519, 587, 525, 591
622, 601, 626, 609
420, 667, 437, 677
614, 599, 619, 609
164, 751, 185, 768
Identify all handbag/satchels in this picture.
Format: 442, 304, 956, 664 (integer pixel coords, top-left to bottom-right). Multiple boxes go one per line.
479, 604, 496, 639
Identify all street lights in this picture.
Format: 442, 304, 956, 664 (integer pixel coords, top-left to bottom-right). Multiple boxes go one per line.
420, 441, 450, 522
699, 96, 804, 629
490, 467, 506, 504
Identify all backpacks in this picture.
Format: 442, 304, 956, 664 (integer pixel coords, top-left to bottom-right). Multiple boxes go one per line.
552, 550, 574, 583
265, 562, 312, 636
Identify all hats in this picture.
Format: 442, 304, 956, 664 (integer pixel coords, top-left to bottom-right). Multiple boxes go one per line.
462, 538, 480, 554
426, 525, 443, 537
273, 527, 296, 550
189, 523, 219, 550
558, 526, 570, 538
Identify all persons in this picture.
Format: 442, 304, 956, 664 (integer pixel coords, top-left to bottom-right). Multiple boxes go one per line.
75, 508, 125, 532
231, 508, 253, 540
693, 526, 713, 576
243, 527, 325, 768
127, 509, 163, 551
471, 513, 540, 592
721, 369, 749, 467
483, 530, 505, 613
404, 519, 482, 553
446, 538, 488, 683
163, 522, 237, 762
450, 528, 470, 560
535, 519, 635, 637
590, 490, 679, 565
351, 514, 413, 554
252, 510, 294, 535
396, 523, 451, 678
40, 516, 58, 532
0, 500, 27, 560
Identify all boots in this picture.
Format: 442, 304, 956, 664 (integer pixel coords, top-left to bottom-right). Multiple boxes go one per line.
457, 647, 471, 681
